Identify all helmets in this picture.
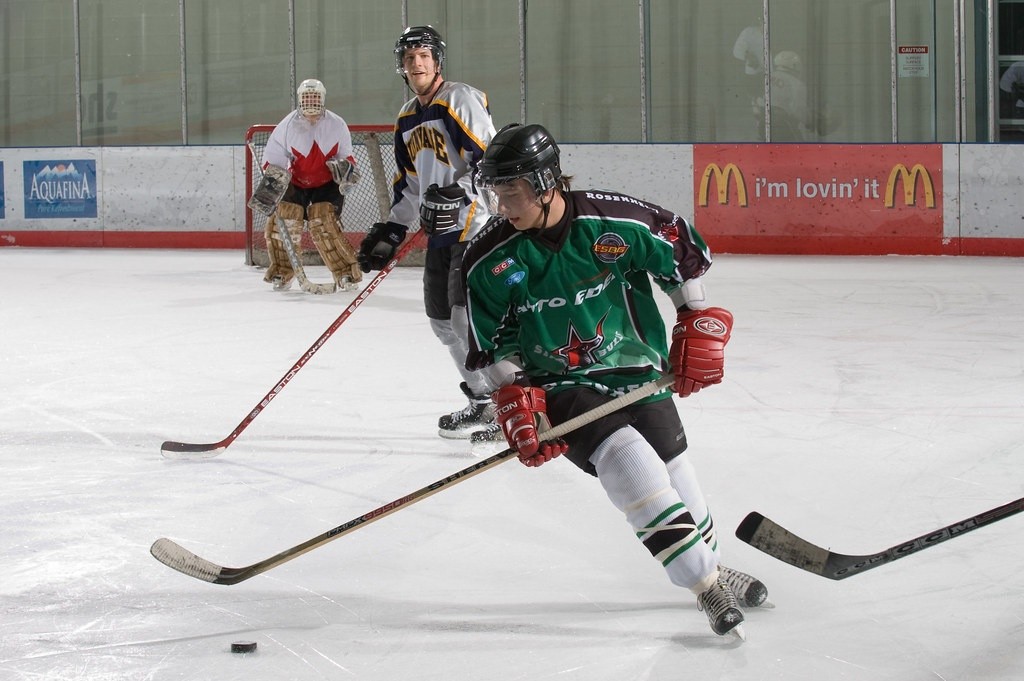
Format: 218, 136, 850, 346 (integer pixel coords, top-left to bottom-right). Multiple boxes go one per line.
478, 122, 563, 192
297, 79, 326, 103
395, 26, 447, 61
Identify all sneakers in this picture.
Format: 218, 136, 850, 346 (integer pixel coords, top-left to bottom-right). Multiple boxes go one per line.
471, 417, 507, 444
438, 382, 498, 439
696, 577, 747, 642
719, 564, 768, 608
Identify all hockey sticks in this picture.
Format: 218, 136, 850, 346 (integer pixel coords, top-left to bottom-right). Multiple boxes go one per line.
737, 488, 1024, 580
246, 136, 338, 293
146, 364, 677, 584
159, 202, 426, 458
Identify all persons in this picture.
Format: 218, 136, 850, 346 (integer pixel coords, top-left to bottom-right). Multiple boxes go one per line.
247, 77, 362, 290
463, 123, 769, 636
354, 21, 510, 440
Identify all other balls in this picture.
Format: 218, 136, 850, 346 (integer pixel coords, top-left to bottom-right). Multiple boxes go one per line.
230, 640, 259, 654
